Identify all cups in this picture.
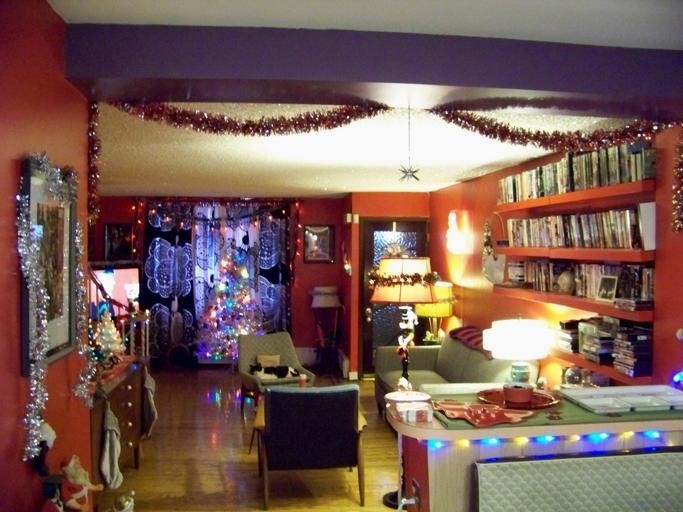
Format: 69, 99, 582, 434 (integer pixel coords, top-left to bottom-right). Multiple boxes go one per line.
299, 374, 306, 386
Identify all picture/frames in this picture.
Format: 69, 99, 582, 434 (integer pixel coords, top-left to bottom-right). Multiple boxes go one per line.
102, 221, 136, 262
19, 154, 82, 380
302, 222, 336, 265
594, 274, 618, 304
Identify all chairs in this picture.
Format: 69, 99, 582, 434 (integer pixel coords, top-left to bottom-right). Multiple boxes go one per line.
245, 382, 367, 510
236, 331, 316, 409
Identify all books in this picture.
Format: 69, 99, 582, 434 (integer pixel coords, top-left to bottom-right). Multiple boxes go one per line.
496, 142, 655, 377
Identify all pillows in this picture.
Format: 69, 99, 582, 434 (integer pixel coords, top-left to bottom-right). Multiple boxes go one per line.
255, 353, 280, 381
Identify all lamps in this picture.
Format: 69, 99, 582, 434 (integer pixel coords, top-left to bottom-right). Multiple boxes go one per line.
473, 317, 561, 411
414, 279, 456, 341
370, 255, 436, 385
311, 283, 343, 377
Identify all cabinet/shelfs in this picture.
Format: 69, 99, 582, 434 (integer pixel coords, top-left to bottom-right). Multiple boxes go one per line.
489, 177, 661, 388
84, 352, 152, 512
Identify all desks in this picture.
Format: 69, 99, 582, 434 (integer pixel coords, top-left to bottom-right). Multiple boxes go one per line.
379, 375, 682, 511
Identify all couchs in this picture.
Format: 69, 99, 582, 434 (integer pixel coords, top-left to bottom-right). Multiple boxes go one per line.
373, 330, 542, 440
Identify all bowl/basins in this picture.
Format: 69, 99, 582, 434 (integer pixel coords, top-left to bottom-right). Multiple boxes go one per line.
384, 391, 432, 411
504, 384, 533, 402
